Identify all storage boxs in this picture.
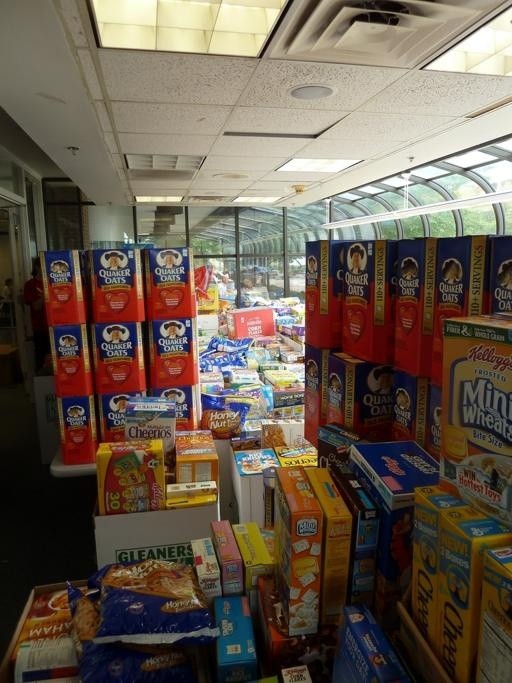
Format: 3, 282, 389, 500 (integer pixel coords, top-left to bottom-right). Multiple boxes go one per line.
92, 484, 221, 570
0, 579, 89, 682
225, 306, 274, 339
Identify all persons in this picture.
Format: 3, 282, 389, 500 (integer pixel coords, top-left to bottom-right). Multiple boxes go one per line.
236, 278, 265, 308
25, 257, 47, 359
219, 269, 237, 299
2, 278, 14, 298
